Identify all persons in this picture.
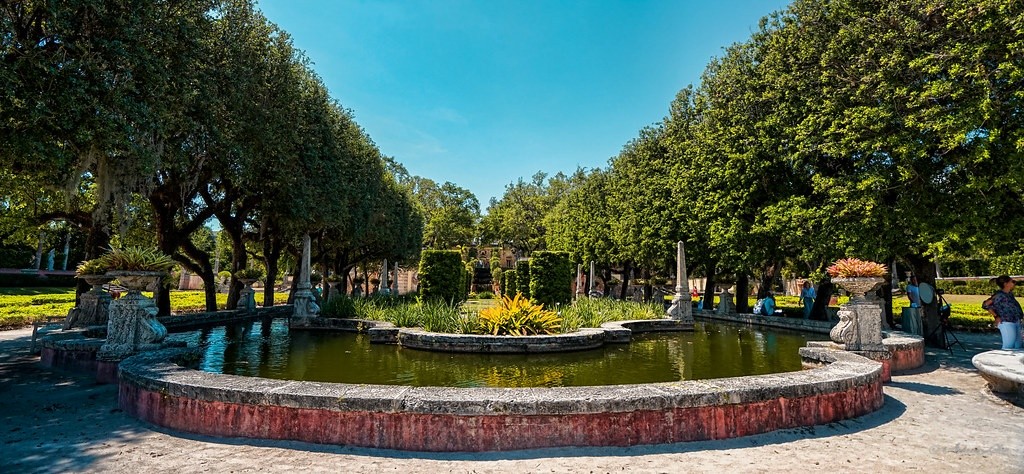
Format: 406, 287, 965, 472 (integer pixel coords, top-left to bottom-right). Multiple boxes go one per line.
982, 276, 1023, 349
799, 281, 816, 320
108, 291, 121, 300
752, 292, 787, 317
698, 297, 703, 311
907, 275, 921, 307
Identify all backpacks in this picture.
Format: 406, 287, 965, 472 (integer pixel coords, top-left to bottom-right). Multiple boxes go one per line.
760, 298, 771, 316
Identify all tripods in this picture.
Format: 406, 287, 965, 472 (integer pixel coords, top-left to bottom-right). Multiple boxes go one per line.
925, 296, 967, 355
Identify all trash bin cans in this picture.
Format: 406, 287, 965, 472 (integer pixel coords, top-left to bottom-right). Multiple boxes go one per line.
902, 307, 922, 335
